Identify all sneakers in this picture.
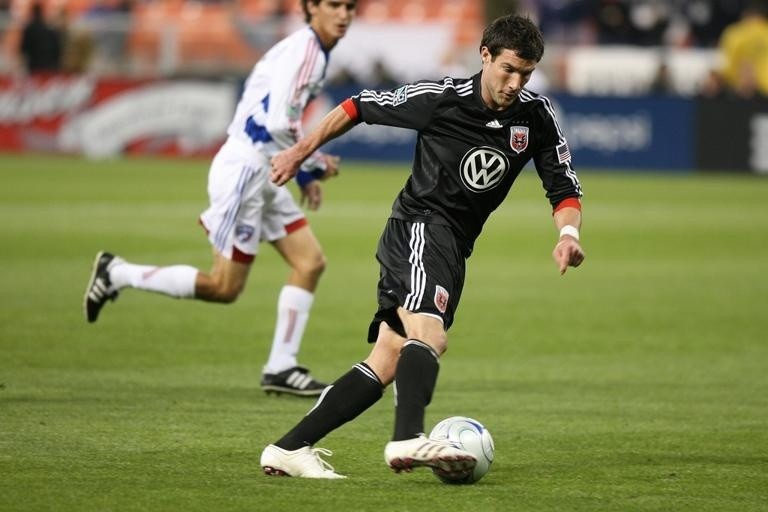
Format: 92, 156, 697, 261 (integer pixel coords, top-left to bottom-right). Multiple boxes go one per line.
258, 363, 332, 397
382, 430, 478, 482
259, 442, 349, 479
82, 249, 119, 323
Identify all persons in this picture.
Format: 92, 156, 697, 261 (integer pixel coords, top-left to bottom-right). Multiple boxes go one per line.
83, 0, 357, 396
260, 14, 585, 479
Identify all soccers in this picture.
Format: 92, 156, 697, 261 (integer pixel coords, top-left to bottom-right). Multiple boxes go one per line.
429, 416, 495, 484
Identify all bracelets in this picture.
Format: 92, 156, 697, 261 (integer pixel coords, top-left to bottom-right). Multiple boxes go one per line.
560, 224, 580, 241
